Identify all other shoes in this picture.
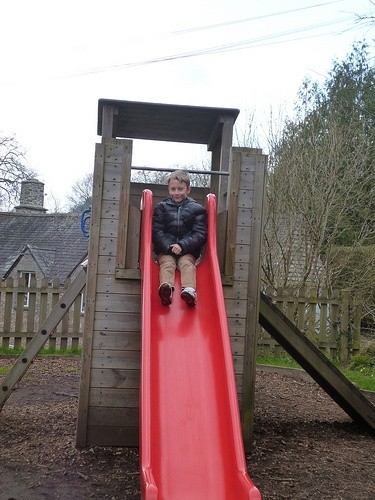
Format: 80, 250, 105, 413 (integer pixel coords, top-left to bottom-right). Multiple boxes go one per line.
181, 287, 197, 306
158, 281, 173, 305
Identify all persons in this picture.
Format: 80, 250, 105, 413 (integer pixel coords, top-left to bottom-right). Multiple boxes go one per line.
150, 169, 207, 307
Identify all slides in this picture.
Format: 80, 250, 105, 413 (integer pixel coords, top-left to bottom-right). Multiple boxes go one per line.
137, 189, 272, 500
